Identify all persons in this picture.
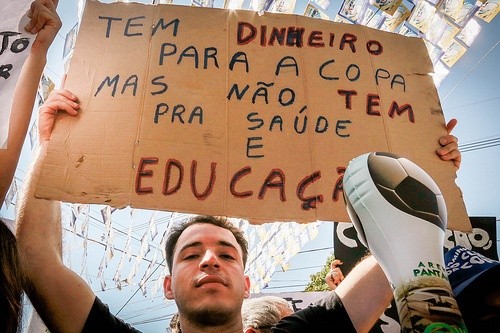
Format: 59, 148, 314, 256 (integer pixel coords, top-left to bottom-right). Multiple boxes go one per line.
242, 259, 345, 333
0, 0, 64, 333
15, 71, 462, 333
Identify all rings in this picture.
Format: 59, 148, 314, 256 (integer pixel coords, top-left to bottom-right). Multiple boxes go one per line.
331, 272, 334, 279
